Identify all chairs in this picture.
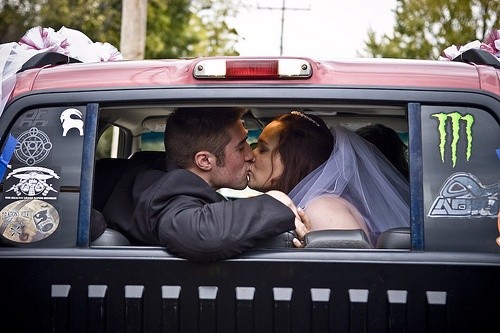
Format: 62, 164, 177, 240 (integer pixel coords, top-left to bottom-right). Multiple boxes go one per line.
89, 149, 166, 247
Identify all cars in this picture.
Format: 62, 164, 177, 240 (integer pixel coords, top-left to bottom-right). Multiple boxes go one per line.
0, 51, 500, 333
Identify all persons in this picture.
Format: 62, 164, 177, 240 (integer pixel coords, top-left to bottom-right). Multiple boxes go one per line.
247, 112, 375, 249
123, 107, 302, 260
